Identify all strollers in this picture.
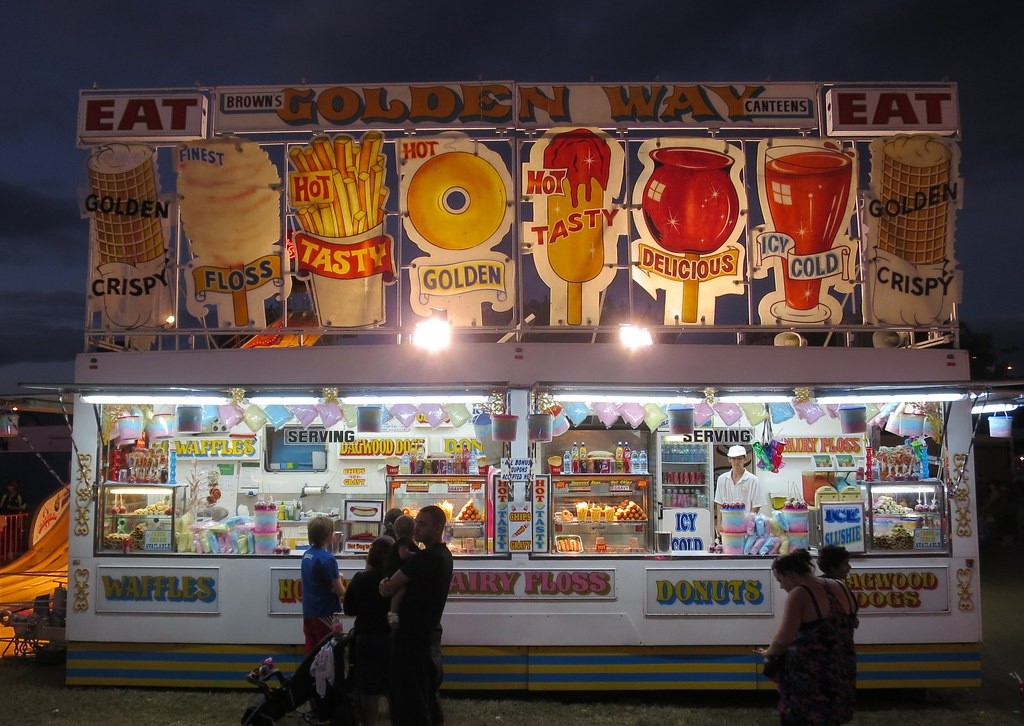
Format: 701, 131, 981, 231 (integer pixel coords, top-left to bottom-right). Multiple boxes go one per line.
240, 628, 352, 726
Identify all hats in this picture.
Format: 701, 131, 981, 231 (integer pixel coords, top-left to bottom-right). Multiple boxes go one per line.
727, 446, 747, 459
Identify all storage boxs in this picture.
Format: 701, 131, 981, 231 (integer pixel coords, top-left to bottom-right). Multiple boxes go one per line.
654, 531, 672, 554
801, 472, 836, 505
772, 496, 787, 509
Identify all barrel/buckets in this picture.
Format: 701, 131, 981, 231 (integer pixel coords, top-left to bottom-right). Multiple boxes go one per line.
117, 415, 142, 440
782, 507, 809, 533
355, 405, 382, 432
837, 394, 867, 434
528, 397, 553, 443
491, 401, 518, 443
667, 393, 693, 434
720, 508, 744, 533
787, 532, 810, 553
721, 534, 745, 557
253, 509, 277, 533
988, 409, 1011, 437
254, 532, 277, 555
176, 404, 203, 433
153, 413, 174, 439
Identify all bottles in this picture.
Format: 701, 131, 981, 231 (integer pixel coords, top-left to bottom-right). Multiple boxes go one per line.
616, 441, 631, 473
453, 445, 478, 474
401, 444, 425, 474
631, 450, 648, 474
662, 441, 707, 462
856, 460, 865, 481
873, 458, 880, 483
563, 441, 587, 473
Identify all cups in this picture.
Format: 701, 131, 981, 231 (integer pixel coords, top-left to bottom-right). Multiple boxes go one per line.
764, 146, 853, 321
590, 507, 602, 522
605, 507, 615, 523
576, 502, 588, 521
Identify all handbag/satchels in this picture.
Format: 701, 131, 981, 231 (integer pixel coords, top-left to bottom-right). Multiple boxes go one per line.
763, 655, 785, 684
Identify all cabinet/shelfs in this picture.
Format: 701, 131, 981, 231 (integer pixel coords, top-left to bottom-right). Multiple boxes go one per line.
868, 481, 944, 550
99, 484, 187, 552
552, 474, 654, 551
386, 474, 489, 553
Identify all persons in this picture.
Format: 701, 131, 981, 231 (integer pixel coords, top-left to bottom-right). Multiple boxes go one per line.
714, 445, 766, 535
0, 479, 26, 514
343, 505, 453, 726
301, 516, 346, 657
752, 544, 860, 726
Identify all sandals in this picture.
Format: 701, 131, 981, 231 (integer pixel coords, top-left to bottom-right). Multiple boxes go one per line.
387, 612, 399, 628
